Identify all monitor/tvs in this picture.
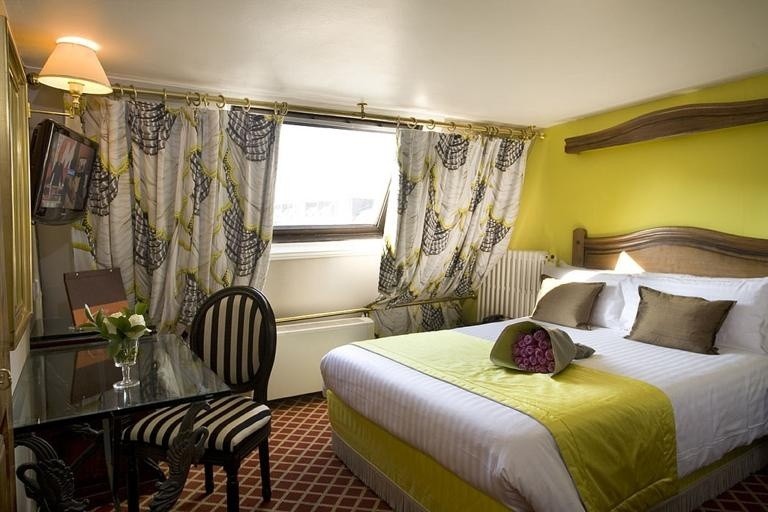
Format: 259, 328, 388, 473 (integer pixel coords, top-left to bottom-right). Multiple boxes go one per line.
29, 117, 100, 225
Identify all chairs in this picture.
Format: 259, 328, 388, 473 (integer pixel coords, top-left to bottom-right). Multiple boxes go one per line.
121, 286, 277, 511
64, 267, 130, 332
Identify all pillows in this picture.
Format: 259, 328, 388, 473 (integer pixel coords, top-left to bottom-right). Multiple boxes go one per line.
530, 250, 768, 355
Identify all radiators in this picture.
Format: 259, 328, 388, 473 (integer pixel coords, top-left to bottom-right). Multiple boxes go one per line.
476, 249, 557, 325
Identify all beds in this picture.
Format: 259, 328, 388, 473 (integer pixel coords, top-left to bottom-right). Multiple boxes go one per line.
318, 224, 768, 512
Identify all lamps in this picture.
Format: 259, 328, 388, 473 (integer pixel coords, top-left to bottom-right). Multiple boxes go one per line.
27, 35, 114, 120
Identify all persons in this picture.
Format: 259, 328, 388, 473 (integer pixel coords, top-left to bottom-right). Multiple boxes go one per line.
49, 158, 63, 190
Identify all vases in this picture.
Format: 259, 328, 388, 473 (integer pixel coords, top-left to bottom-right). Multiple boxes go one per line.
106, 337, 141, 389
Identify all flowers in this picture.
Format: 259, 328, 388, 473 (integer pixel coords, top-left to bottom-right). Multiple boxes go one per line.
75, 303, 152, 362
490, 320, 595, 377
